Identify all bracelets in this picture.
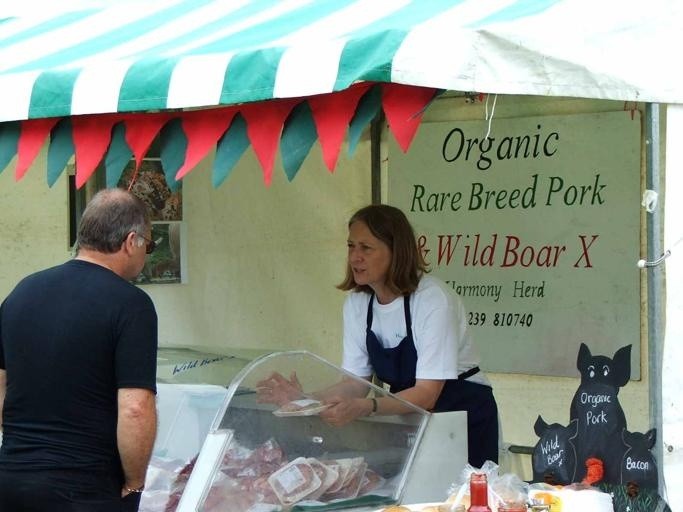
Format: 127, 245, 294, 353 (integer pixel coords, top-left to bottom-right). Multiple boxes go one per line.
125, 483, 144, 494
368, 396, 378, 416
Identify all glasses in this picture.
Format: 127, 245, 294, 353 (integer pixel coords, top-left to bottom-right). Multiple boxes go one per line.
138, 231, 156, 255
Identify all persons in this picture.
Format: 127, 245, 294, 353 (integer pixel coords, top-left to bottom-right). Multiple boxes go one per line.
0, 187, 159, 511
254, 204, 507, 474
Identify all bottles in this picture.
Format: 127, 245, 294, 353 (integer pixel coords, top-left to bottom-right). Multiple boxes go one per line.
467, 472, 493, 512
